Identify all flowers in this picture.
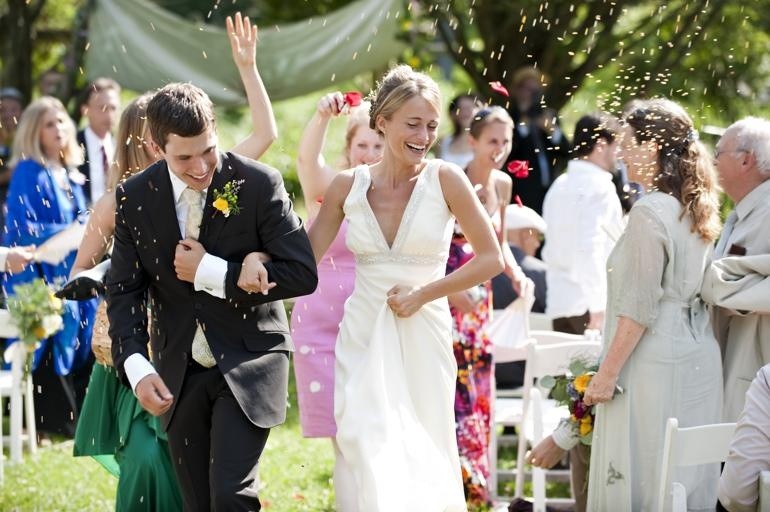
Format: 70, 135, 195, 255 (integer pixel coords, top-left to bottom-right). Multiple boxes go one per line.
207, 178, 246, 220
539, 358, 622, 436
6, 277, 64, 382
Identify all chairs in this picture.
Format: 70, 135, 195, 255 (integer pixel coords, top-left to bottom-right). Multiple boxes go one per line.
486, 309, 552, 398
530, 341, 606, 512
486, 341, 531, 504
0, 310, 41, 464
657, 417, 737, 512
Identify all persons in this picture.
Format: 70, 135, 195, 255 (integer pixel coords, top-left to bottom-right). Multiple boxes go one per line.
581, 103, 723, 512
439, 89, 488, 174
109, 83, 318, 512
716, 358, 770, 512
1, 245, 33, 275
35, 66, 67, 99
292, 92, 384, 512
446, 105, 533, 512
0, 87, 20, 232
1, 96, 91, 435
612, 147, 645, 211
236, 65, 504, 512
73, 76, 123, 204
541, 111, 623, 335
67, 10, 280, 512
510, 67, 571, 202
524, 411, 593, 509
708, 114, 770, 258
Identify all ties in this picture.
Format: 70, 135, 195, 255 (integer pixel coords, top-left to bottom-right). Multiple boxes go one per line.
101, 147, 114, 176
184, 187, 219, 367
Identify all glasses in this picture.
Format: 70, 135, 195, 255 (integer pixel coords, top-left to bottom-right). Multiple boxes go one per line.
716, 147, 751, 159
